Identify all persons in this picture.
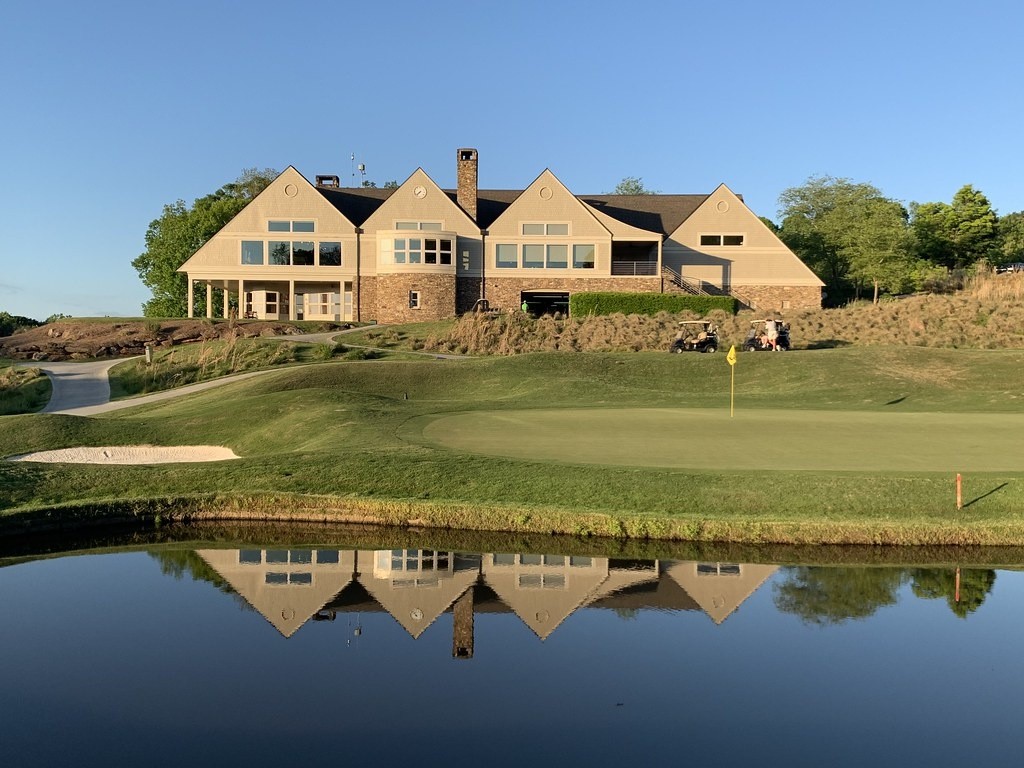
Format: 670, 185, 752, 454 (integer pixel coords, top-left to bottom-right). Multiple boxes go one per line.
765, 319, 781, 351
761, 334, 769, 348
521, 300, 529, 313
